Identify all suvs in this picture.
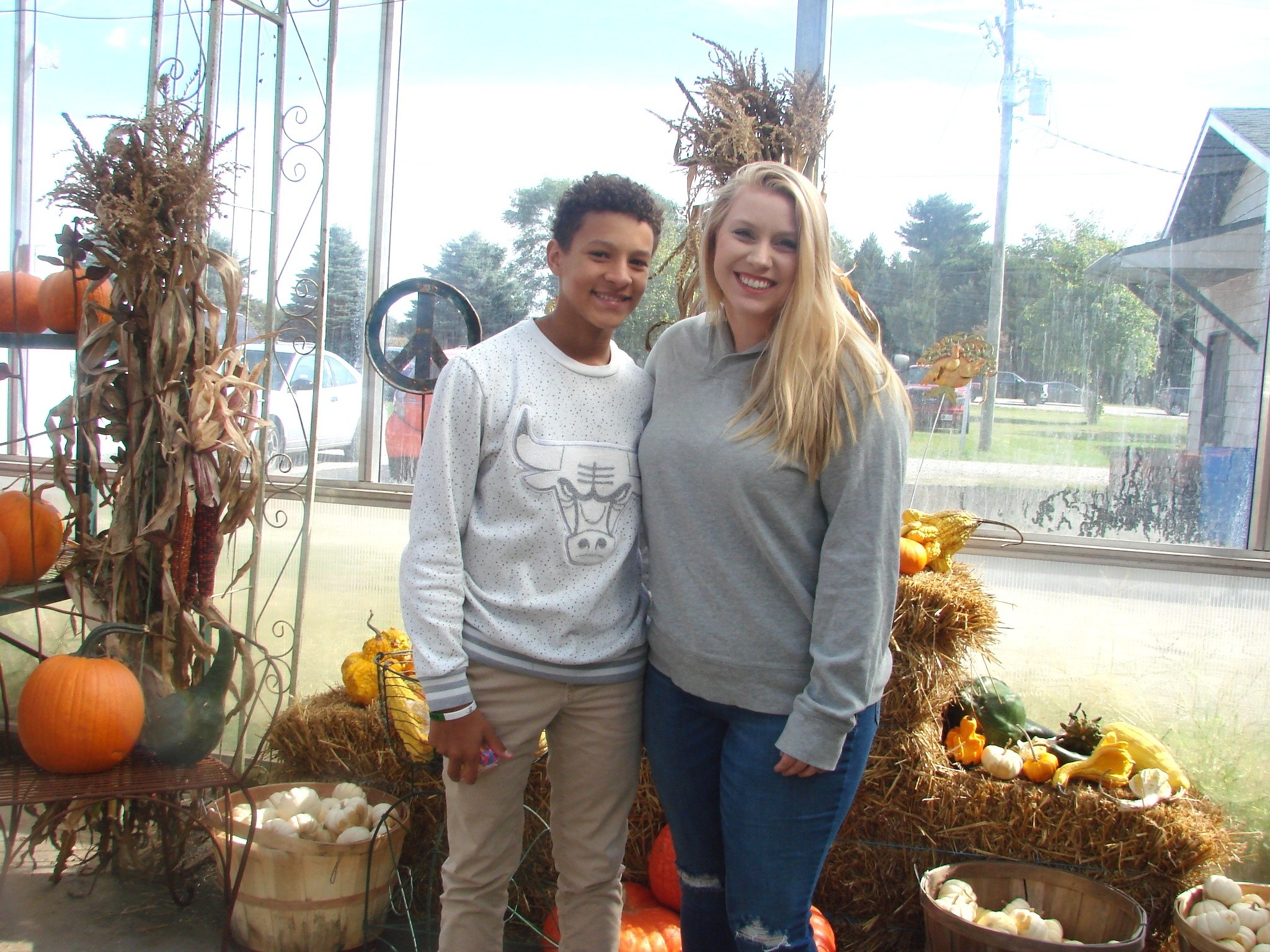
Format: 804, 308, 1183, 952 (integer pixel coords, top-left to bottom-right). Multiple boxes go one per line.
970, 372, 1046, 406
1156, 388, 1190, 416
1042, 383, 1104, 408
902, 364, 983, 435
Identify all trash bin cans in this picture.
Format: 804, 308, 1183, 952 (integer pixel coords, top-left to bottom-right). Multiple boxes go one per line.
1198, 445, 1256, 551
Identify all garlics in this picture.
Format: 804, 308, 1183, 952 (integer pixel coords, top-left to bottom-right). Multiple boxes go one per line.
935, 879, 1121, 944
218, 782, 399, 844
1185, 874, 1270, 952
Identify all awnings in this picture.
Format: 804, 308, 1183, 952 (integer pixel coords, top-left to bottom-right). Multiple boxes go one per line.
1088, 215, 1266, 357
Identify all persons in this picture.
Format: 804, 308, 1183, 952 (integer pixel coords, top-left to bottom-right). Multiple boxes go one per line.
646, 160, 913, 951
396, 169, 666, 952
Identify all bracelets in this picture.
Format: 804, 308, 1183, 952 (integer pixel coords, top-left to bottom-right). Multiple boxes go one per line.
430, 699, 477, 721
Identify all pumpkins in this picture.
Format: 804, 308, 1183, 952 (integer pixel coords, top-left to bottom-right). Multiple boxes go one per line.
341, 609, 548, 763
0, 244, 234, 772
900, 507, 1190, 812
543, 823, 835, 952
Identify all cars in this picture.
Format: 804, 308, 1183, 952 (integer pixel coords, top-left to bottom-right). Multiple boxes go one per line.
387, 347, 467, 480
221, 343, 367, 469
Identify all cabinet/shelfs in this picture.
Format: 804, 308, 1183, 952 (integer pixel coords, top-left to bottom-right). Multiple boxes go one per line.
0, 333, 98, 659
